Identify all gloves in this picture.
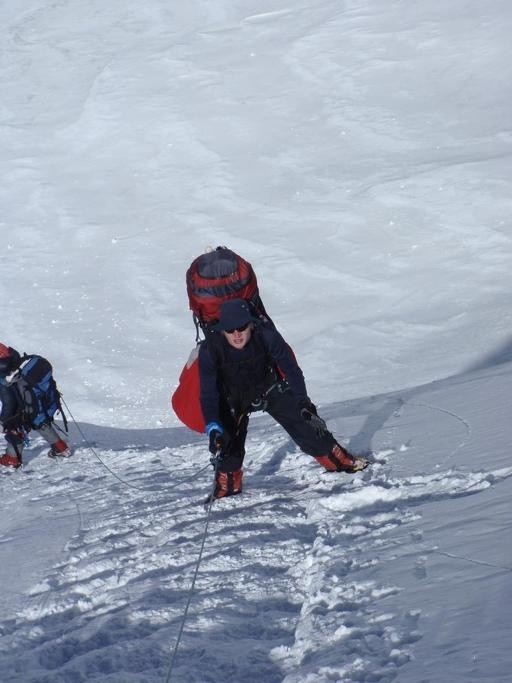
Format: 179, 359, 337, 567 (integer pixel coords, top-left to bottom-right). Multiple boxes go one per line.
209, 429, 231, 457
299, 409, 326, 438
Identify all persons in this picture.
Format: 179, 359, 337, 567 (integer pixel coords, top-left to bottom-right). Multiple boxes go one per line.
0, 343, 72, 471
197, 298, 370, 504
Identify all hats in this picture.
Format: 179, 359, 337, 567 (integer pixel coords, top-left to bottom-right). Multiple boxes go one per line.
211, 298, 258, 331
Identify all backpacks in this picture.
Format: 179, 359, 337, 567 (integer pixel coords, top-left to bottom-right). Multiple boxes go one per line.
0, 352, 68, 434
186, 246, 262, 343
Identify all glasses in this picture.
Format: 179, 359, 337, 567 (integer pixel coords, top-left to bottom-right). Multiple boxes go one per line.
225, 324, 249, 333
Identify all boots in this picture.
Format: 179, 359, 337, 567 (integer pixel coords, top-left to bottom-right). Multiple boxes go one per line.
0, 454, 22, 468
47, 440, 71, 457
316, 441, 368, 473
206, 471, 242, 504
